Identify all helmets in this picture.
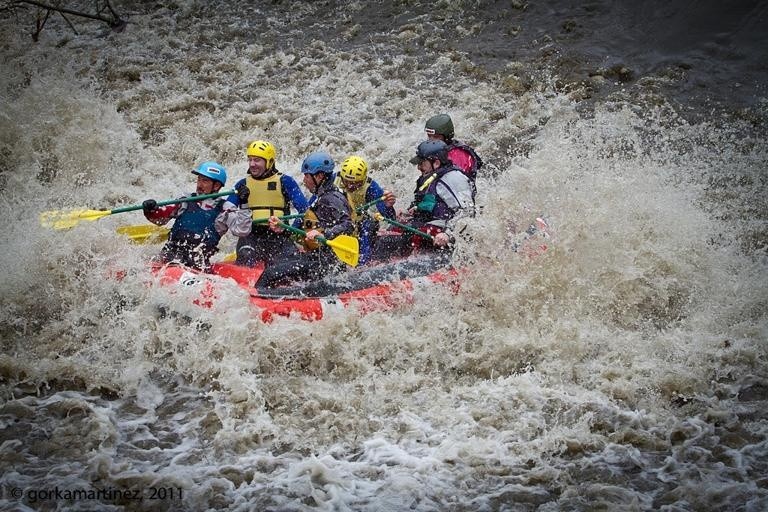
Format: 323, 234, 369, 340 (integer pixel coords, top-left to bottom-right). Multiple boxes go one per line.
424, 114, 454, 139
247, 140, 276, 169
340, 157, 368, 185
191, 162, 227, 187
301, 152, 334, 175
416, 140, 449, 165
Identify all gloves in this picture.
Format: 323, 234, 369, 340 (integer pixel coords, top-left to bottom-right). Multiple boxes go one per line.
238, 184, 251, 204
143, 200, 157, 214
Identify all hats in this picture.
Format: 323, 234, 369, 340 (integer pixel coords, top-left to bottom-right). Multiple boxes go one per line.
409, 156, 424, 165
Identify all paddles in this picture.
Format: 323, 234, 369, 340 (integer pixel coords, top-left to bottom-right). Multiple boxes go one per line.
40, 184, 239, 229
269, 217, 359, 268
112, 213, 305, 243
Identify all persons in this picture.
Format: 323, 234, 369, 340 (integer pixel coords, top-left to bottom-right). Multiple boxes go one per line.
424, 114, 482, 179
333, 156, 396, 269
226, 140, 312, 269
253, 152, 356, 287
395, 140, 478, 258
143, 161, 254, 273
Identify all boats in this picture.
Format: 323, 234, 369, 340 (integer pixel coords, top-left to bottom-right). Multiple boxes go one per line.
106, 218, 550, 347
